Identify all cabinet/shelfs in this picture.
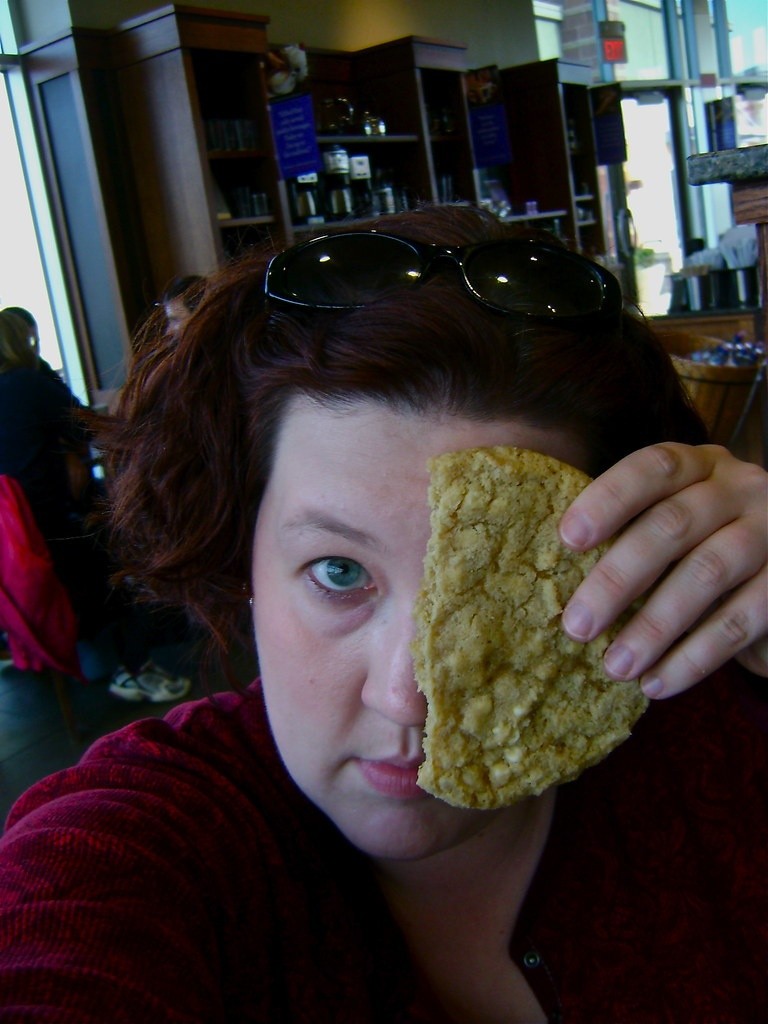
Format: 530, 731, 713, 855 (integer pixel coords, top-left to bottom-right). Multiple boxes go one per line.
293, 134, 423, 235
102, 4, 293, 302
496, 57, 609, 256
353, 35, 483, 209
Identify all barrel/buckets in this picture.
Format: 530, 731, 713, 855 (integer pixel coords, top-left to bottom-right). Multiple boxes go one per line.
668, 334, 766, 450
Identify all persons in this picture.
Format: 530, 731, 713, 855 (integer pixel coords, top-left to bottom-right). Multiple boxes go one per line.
1, 307, 191, 702
0, 203, 768, 1023
164, 271, 207, 339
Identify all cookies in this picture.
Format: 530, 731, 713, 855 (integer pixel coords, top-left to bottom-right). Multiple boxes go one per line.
409, 448, 654, 811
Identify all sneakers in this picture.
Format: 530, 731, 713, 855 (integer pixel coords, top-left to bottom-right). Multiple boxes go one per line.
110, 664, 192, 701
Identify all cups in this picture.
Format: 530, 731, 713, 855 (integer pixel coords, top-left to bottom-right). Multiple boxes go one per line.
526, 200, 538, 215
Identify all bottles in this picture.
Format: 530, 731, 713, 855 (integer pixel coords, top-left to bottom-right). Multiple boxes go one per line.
692, 331, 766, 368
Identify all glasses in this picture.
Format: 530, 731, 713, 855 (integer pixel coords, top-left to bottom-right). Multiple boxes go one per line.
223, 225, 623, 373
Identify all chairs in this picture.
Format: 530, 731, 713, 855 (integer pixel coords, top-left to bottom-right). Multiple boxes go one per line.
0, 475, 125, 746
